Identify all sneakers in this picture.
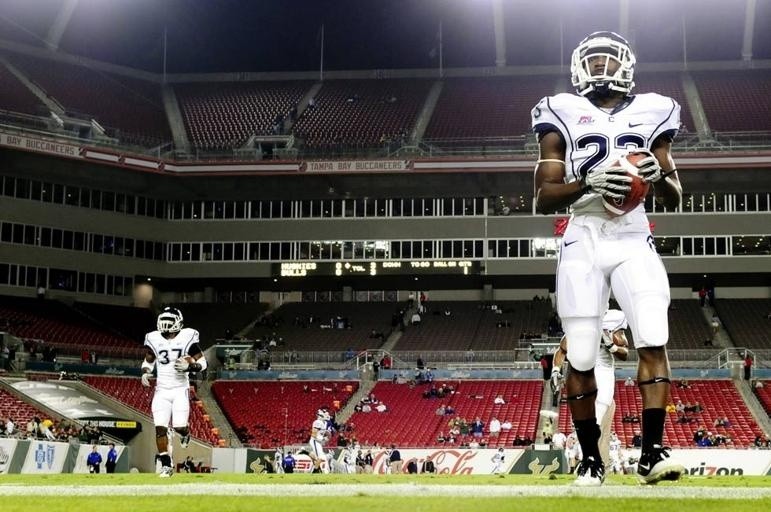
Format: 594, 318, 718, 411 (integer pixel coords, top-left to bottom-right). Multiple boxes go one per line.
159, 466, 175, 479
635, 448, 684, 484
572, 457, 606, 489
180, 434, 191, 449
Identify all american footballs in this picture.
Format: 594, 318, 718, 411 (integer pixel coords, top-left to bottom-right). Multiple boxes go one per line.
603, 152, 651, 218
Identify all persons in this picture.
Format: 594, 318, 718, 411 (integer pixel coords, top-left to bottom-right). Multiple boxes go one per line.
492, 448, 505, 473
354, 355, 455, 415
263, 451, 296, 473
309, 409, 332, 473
550, 302, 630, 470
257, 354, 270, 371
324, 422, 435, 473
308, 96, 315, 110
495, 395, 505, 404
531, 29, 685, 488
353, 93, 359, 103
7, 338, 98, 373
279, 116, 285, 135
141, 306, 208, 473
480, 295, 563, 361
183, 456, 193, 472
254, 291, 426, 348
287, 105, 298, 124
551, 354, 769, 473
3, 414, 117, 473
437, 416, 534, 448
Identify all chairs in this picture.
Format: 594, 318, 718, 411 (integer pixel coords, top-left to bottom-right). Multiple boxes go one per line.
0, 300, 771, 447
0, 30, 586, 154
634, 33, 768, 151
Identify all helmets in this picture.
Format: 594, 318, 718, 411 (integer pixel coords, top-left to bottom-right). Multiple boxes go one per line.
156, 306, 185, 337
317, 407, 332, 421
569, 30, 637, 96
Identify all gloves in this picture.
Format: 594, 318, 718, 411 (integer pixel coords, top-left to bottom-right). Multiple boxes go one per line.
140, 373, 154, 388
633, 146, 666, 185
602, 330, 618, 354
584, 162, 634, 200
549, 367, 562, 396
173, 355, 190, 374
